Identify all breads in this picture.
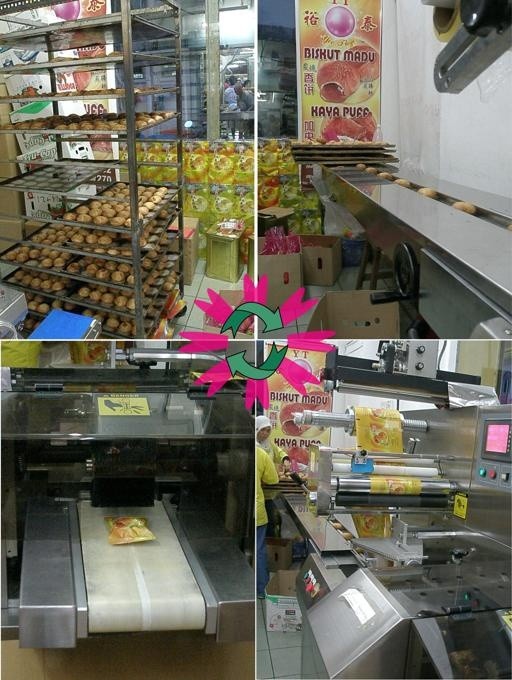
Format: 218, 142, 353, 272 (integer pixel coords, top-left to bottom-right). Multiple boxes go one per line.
6, 222, 134, 259
142, 253, 173, 318
0, 244, 135, 295
333, 523, 341, 529
416, 187, 439, 197
25, 291, 76, 314
279, 403, 313, 431
134, 111, 176, 129
1, 88, 126, 97
452, 200, 478, 214
140, 232, 169, 281
324, 118, 367, 142
64, 182, 167, 228
291, 137, 386, 146
342, 533, 351, 539
344, 46, 380, 82
356, 162, 366, 171
133, 88, 163, 93
365, 167, 378, 175
145, 271, 178, 332
22, 318, 42, 332
78, 283, 134, 315
304, 390, 332, 414
287, 446, 308, 465
0, 110, 124, 131
356, 547, 363, 553
81, 309, 137, 337
140, 210, 167, 248
352, 115, 377, 140
377, 172, 393, 181
394, 179, 410, 187
317, 60, 360, 99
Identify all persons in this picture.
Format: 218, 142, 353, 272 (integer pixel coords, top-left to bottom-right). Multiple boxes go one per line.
221, 72, 255, 139
256, 413, 292, 474
1, 339, 99, 460
256, 446, 279, 599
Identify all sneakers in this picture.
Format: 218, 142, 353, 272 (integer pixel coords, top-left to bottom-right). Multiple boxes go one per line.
257, 591, 265, 600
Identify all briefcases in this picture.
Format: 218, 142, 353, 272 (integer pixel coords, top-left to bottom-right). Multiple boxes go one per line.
293, 540, 310, 561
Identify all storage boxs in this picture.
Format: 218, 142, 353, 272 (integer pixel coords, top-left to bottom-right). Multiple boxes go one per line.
0, 0, 99, 255
262, 567, 308, 634
260, 535, 294, 570
166, 217, 200, 286
255, 228, 400, 341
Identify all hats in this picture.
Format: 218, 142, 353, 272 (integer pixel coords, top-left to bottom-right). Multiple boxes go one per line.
256, 416, 272, 437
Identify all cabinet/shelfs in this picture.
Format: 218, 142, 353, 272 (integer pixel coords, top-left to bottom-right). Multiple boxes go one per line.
0, 0, 186, 340
